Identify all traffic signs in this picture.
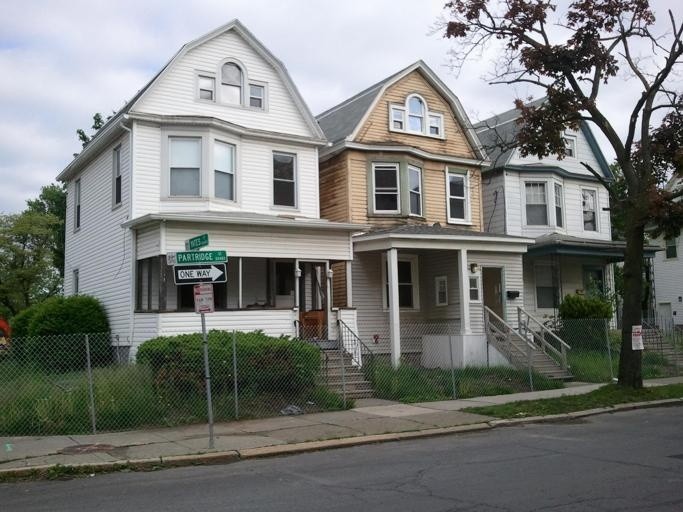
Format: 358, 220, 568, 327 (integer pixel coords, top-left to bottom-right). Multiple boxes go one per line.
167, 251, 229, 266
185, 232, 209, 251
193, 283, 215, 314
172, 263, 228, 284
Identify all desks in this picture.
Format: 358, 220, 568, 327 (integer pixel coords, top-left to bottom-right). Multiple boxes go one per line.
298, 309, 325, 341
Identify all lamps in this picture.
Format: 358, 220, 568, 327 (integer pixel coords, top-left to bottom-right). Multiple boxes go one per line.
294, 267, 302, 278
327, 269, 334, 279
470, 263, 478, 273
